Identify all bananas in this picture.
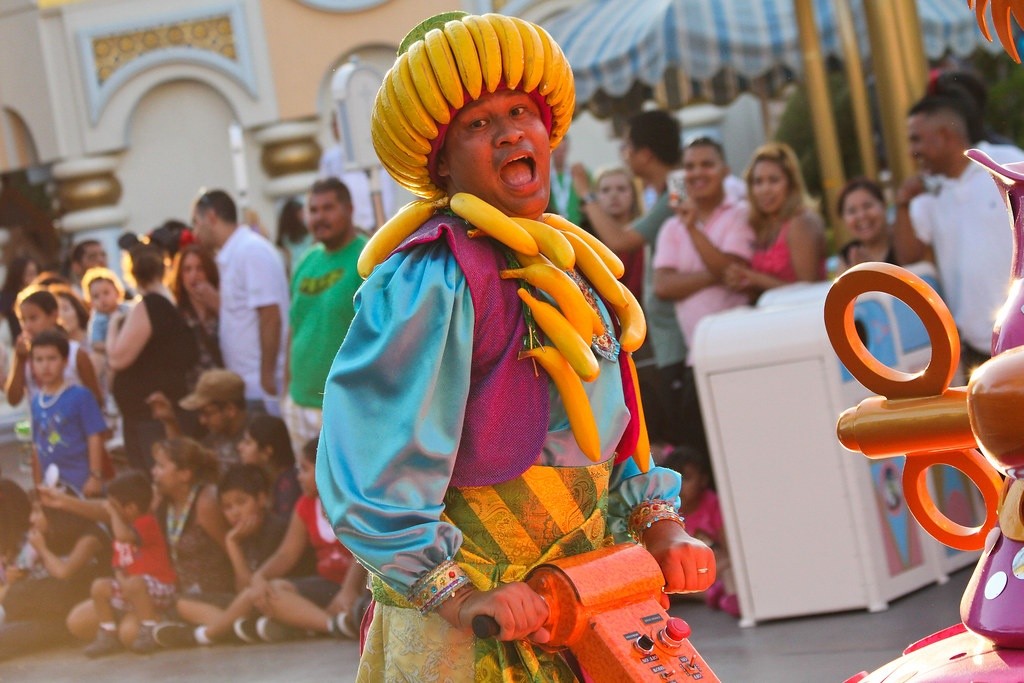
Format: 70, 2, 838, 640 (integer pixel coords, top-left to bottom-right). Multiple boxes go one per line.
356, 192, 650, 474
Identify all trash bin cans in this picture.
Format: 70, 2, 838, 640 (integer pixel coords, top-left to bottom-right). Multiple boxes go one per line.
757, 263, 989, 572
693, 297, 941, 628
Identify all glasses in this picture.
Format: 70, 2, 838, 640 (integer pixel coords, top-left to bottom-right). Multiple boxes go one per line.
199, 409, 219, 416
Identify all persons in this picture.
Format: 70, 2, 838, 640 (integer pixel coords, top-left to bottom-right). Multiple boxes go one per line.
315, 10, 716, 683
547, 75, 1024, 614
0, 176, 371, 658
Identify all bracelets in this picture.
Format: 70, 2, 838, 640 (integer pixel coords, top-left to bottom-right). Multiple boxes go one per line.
580, 195, 597, 206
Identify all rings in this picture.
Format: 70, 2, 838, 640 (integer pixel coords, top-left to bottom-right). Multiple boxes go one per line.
698, 568, 708, 574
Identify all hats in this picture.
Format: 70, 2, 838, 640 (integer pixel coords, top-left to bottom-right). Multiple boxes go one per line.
178, 369, 245, 411
372, 11, 575, 201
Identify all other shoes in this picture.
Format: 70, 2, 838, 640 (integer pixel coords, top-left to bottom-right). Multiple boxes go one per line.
130, 625, 158, 650
156, 624, 198, 647
84, 627, 124, 655
256, 617, 283, 639
333, 611, 359, 640
719, 594, 739, 615
234, 618, 261, 644
706, 580, 722, 606
